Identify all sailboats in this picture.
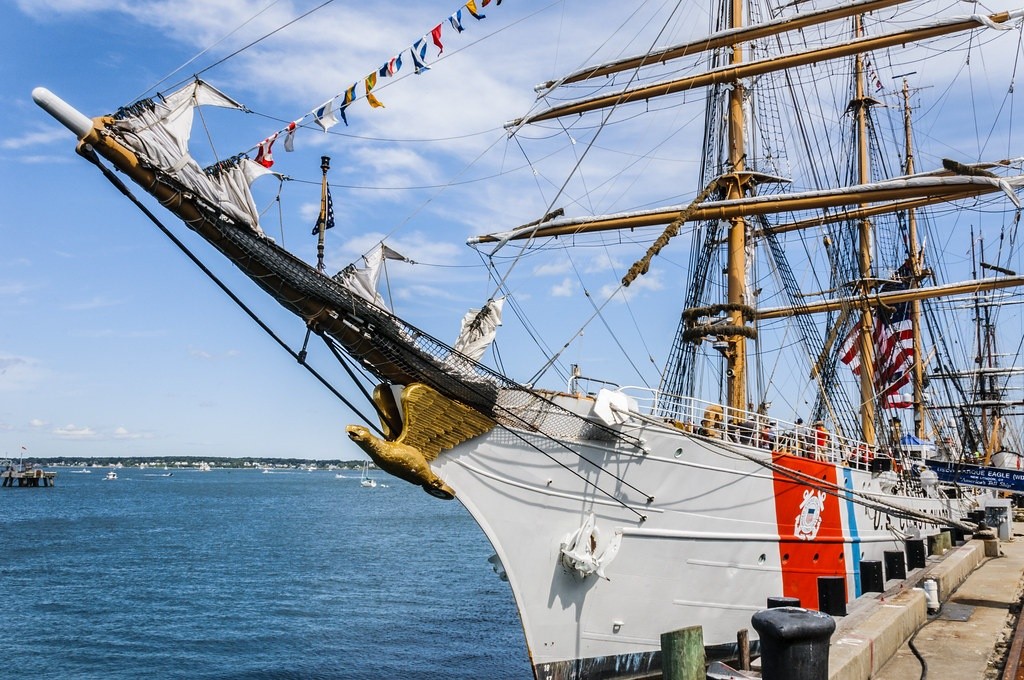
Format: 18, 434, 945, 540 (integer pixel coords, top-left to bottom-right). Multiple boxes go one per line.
30, 1, 1024, 679
358, 459, 376, 488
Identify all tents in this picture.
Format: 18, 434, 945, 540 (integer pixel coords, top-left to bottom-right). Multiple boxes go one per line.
900, 432, 933, 445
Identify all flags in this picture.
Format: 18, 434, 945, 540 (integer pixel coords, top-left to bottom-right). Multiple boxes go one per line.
379, 52, 402, 78
312, 181, 336, 235
364, 72, 385, 109
835, 239, 928, 409
255, 132, 279, 169
483, 0, 503, 6
284, 121, 298, 153
465, 0, 486, 21
409, 35, 432, 76
431, 23, 444, 58
448, 9, 465, 34
312, 99, 340, 133
340, 80, 357, 128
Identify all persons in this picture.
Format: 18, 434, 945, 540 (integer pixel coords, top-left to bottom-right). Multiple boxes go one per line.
666, 411, 779, 451
783, 417, 838, 463
